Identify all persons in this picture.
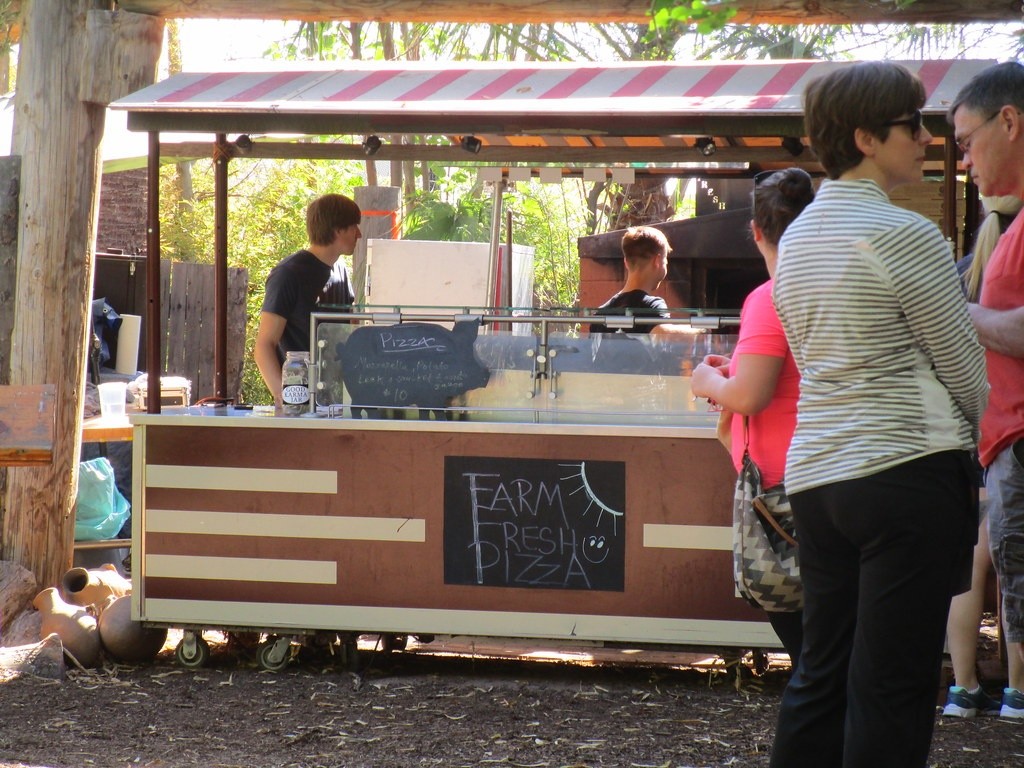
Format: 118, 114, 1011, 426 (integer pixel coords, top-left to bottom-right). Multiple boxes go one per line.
589, 225, 712, 334
255, 195, 362, 418
692, 61, 1024, 768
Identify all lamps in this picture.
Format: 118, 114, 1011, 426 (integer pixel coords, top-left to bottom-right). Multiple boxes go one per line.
361, 134, 381, 156
779, 136, 805, 157
695, 137, 716, 155
234, 134, 253, 156
458, 134, 483, 153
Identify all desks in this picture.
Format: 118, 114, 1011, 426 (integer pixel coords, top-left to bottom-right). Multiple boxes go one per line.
72, 409, 135, 551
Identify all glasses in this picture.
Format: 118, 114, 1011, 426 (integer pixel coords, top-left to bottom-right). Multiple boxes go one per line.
754, 170, 780, 188
882, 110, 922, 141
957, 110, 1021, 156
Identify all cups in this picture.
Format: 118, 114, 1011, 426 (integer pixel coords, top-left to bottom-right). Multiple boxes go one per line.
97, 382, 125, 421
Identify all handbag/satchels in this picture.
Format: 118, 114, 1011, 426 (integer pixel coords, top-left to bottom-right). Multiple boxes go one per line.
732, 459, 811, 615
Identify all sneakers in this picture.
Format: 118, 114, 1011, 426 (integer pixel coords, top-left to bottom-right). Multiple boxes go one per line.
999, 688, 1024, 720
942, 684, 1002, 718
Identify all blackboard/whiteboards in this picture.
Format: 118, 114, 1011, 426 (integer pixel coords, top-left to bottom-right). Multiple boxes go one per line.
441, 451, 628, 592
335, 319, 489, 420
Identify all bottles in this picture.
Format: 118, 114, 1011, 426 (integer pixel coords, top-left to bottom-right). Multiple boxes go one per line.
282, 351, 309, 415
